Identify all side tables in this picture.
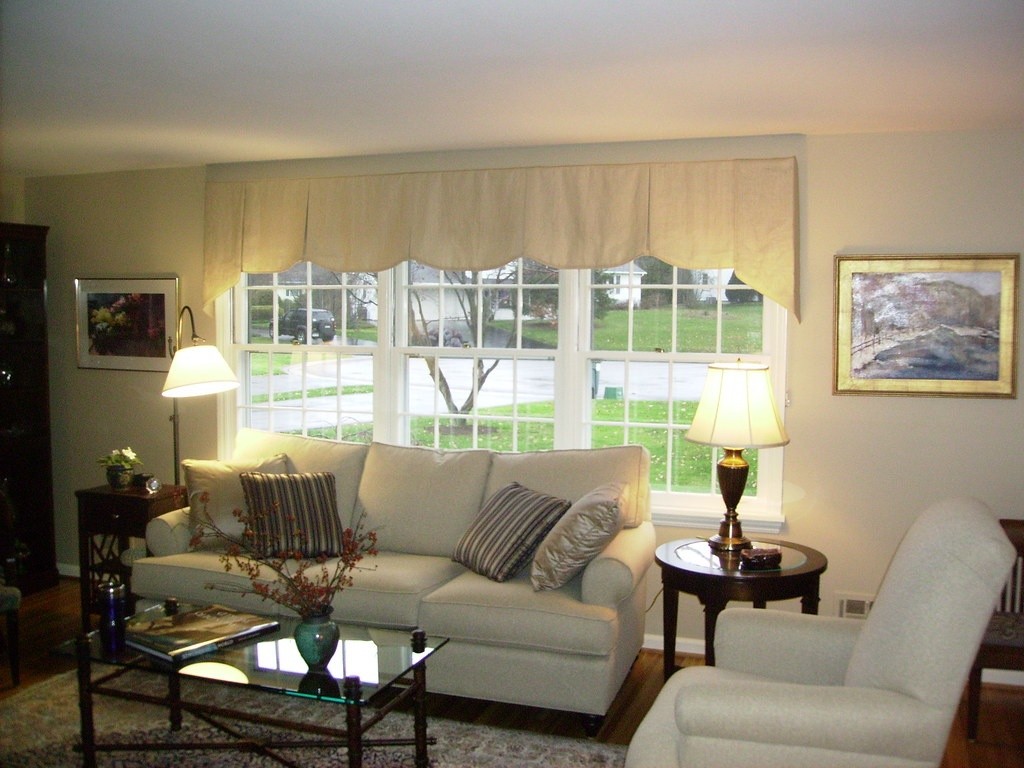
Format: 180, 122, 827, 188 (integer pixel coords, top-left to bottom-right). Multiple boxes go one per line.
651, 534, 829, 679
74, 482, 189, 626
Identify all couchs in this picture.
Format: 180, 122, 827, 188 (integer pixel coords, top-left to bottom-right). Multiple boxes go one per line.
626, 494, 1018, 768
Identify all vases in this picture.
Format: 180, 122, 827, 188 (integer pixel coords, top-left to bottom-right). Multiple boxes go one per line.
290, 603, 341, 670
106, 467, 135, 490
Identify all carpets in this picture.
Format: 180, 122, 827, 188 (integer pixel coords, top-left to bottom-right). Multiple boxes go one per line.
0, 658, 628, 768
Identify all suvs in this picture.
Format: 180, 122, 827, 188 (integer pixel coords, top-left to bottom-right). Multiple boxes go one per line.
268, 307, 336, 343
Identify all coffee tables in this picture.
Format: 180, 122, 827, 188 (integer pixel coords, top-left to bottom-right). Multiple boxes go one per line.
54, 596, 451, 768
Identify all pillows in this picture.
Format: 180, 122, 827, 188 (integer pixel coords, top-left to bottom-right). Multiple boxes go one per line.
130, 428, 661, 736
447, 482, 572, 577
530, 479, 632, 591
182, 454, 290, 549
238, 465, 342, 566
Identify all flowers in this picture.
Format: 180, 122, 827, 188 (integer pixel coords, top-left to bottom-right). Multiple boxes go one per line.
167, 493, 381, 611
95, 446, 143, 468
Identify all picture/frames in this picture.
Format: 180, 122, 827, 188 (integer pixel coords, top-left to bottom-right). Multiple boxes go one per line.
70, 276, 177, 378
830, 253, 1021, 400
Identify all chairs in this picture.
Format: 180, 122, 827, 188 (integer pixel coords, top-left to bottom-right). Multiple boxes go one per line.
964, 519, 1024, 743
0, 486, 23, 688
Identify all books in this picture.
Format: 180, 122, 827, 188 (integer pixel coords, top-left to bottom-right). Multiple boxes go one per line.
124, 602, 280, 663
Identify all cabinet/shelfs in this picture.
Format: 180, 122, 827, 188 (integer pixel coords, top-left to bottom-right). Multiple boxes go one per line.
0, 223, 63, 594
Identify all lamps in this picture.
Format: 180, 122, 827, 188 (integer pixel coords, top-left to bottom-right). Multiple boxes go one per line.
684, 358, 787, 553
162, 304, 243, 481
